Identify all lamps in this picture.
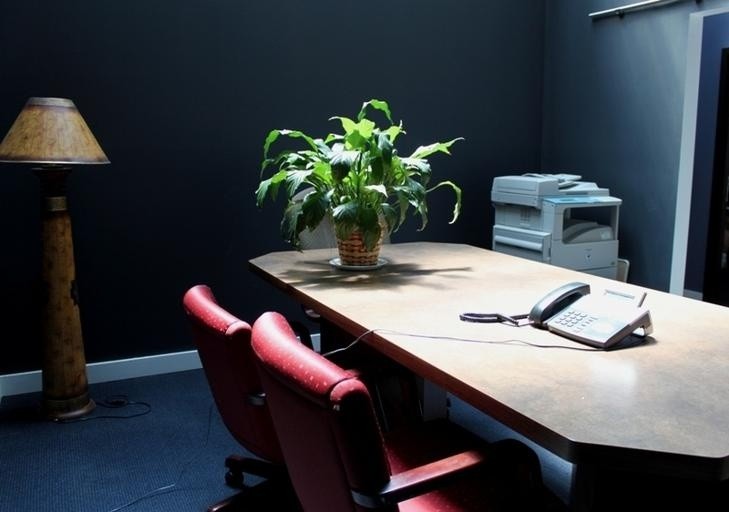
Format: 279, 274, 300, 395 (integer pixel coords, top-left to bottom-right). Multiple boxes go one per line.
1, 97, 112, 421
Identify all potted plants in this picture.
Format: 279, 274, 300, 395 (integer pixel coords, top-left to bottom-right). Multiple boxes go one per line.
252, 98, 465, 266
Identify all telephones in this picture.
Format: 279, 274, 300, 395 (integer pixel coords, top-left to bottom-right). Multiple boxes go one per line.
529, 282, 653, 348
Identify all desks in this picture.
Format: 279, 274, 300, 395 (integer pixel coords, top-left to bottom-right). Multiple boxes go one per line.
248, 240, 729, 512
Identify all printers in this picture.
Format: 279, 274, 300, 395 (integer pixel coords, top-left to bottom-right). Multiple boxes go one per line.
491, 173, 623, 280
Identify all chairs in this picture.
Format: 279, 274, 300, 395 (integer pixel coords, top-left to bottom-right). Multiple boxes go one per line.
295, 208, 392, 323
183, 283, 300, 511
249, 284, 540, 512
